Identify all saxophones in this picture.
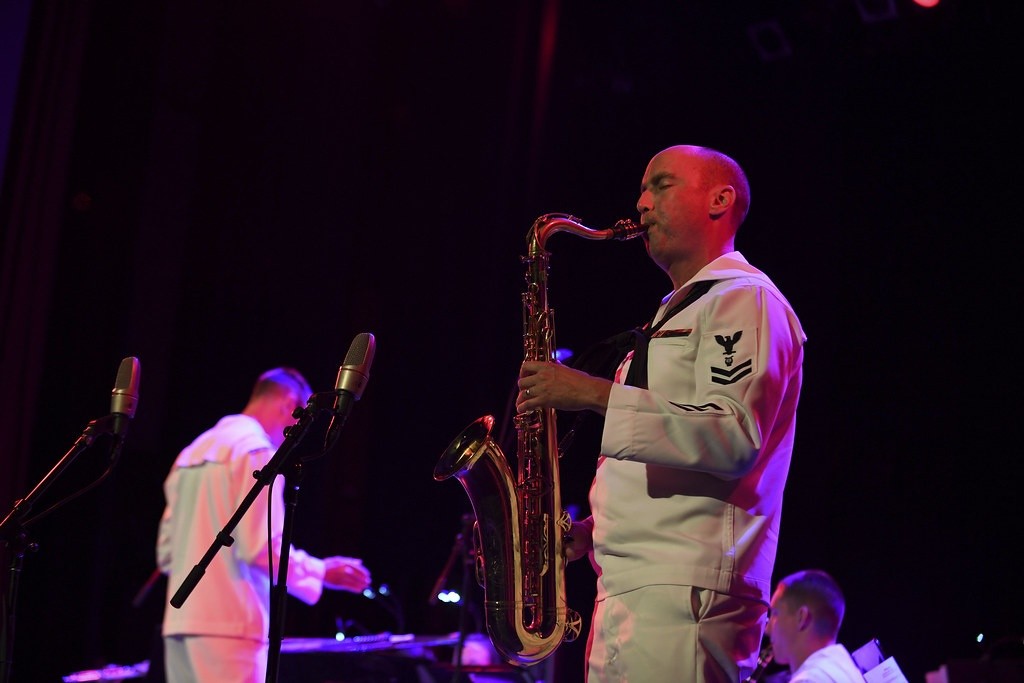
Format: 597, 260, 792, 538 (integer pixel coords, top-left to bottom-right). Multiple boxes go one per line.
431, 212, 647, 668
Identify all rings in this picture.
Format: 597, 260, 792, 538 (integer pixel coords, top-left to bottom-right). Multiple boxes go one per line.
526, 389, 530, 397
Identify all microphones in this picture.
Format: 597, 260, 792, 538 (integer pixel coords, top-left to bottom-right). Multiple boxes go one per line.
110, 357, 142, 435
326, 333, 376, 441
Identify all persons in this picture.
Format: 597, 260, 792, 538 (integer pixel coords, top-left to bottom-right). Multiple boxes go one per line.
517, 145, 807, 683
766, 570, 866, 683
158, 368, 371, 683
